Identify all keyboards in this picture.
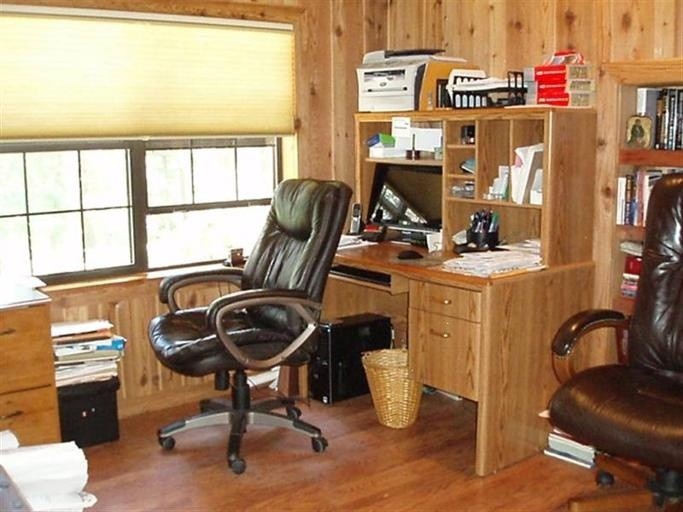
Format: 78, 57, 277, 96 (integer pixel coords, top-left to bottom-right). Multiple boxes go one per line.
331, 265, 391, 287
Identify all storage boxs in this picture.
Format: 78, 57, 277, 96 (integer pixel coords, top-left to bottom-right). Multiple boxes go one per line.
57, 377, 121, 447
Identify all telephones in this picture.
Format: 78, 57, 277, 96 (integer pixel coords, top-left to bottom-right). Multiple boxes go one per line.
346, 201, 364, 235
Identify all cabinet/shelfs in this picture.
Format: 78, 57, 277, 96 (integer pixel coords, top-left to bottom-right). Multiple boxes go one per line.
300, 103, 598, 477
608, 61, 683, 322
0, 298, 59, 448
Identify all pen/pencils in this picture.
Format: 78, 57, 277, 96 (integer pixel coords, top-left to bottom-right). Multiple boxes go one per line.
471, 209, 500, 233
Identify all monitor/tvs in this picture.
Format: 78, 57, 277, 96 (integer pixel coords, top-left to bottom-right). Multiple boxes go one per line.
367, 163, 442, 247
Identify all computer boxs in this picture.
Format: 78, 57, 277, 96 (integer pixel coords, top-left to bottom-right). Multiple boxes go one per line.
310, 312, 391, 405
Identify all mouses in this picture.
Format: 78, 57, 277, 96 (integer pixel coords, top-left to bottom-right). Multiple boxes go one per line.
398, 249, 422, 262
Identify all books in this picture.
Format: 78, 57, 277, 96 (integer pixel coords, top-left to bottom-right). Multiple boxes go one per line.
527, 78, 592, 93
616, 168, 679, 228
49, 318, 126, 389
637, 86, 682, 150
526, 93, 590, 108
516, 147, 544, 205
523, 64, 594, 82
544, 431, 599, 470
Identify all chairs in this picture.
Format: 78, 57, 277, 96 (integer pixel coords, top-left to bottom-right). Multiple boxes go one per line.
148, 178, 353, 475
548, 172, 683, 511
